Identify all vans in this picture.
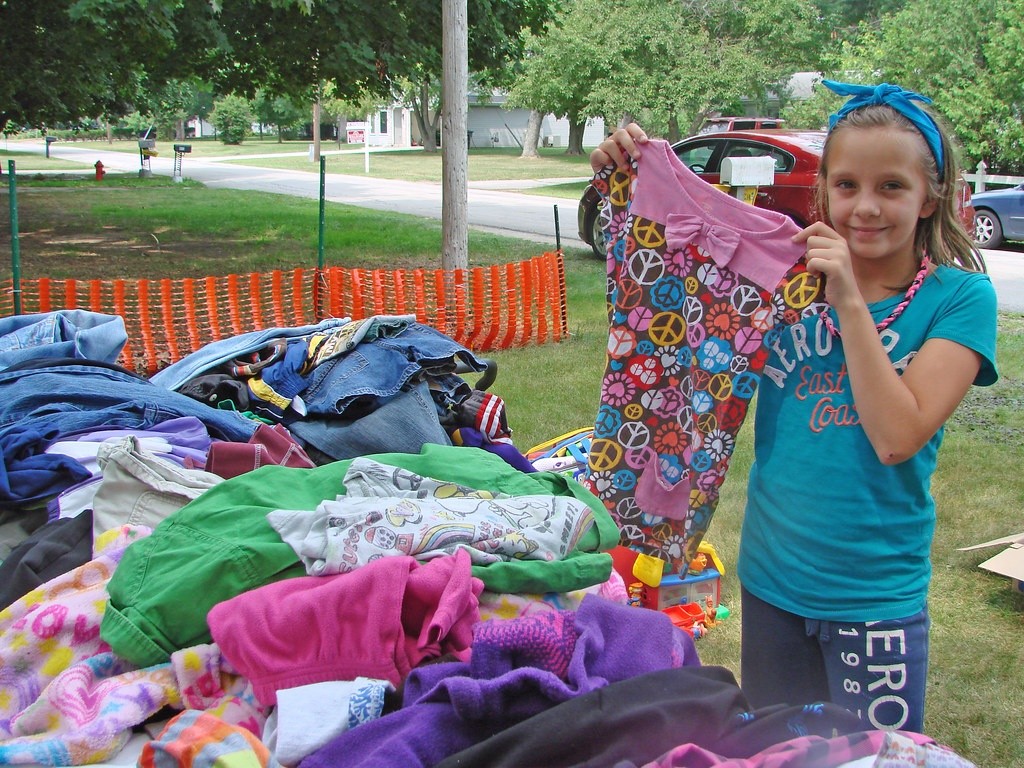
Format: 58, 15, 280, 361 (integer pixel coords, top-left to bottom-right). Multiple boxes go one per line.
687, 114, 786, 175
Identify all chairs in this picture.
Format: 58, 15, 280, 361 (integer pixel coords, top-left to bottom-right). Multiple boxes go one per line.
730, 150, 752, 157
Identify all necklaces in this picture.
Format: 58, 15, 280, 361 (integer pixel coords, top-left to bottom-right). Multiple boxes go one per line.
819, 249, 928, 339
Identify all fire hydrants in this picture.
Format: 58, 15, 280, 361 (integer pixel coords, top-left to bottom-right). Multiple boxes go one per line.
94, 160, 105, 181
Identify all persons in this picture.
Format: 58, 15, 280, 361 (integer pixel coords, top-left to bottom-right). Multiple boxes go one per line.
590, 80, 1000, 733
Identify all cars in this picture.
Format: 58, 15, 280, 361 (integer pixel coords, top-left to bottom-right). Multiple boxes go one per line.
960, 179, 1024, 250
577, 128, 836, 262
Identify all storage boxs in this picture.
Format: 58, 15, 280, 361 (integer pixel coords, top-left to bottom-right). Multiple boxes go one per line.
958, 532, 1024, 595
